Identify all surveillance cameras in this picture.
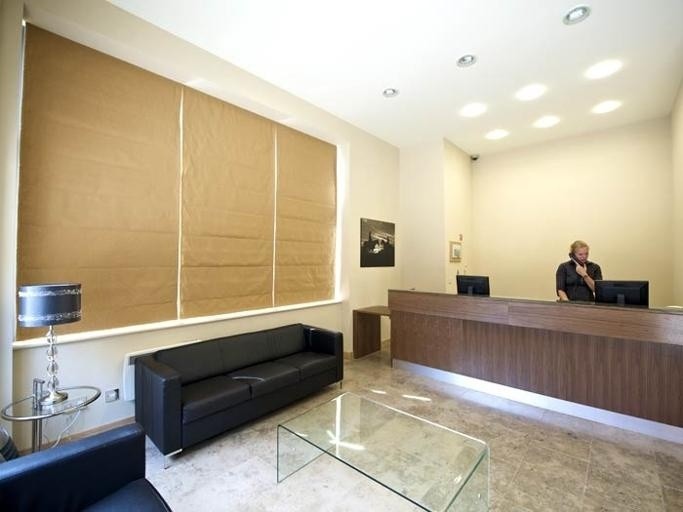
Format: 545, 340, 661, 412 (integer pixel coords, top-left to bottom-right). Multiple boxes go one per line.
471, 156, 478, 160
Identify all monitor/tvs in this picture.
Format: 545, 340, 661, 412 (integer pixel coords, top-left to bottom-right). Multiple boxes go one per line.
595, 280, 648, 308
457, 275, 489, 296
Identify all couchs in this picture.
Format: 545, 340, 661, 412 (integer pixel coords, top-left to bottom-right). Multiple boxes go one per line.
134, 323, 346, 456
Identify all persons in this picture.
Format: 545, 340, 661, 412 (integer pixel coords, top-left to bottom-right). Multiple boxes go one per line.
556, 241, 602, 301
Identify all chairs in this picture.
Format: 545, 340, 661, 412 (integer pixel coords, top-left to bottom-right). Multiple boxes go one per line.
1, 417, 174, 511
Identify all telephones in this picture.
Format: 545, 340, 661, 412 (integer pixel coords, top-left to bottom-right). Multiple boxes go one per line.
569, 253, 584, 266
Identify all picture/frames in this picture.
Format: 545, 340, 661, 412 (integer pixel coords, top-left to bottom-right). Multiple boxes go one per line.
449, 240, 463, 263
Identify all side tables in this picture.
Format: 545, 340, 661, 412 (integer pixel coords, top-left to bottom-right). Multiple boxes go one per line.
2, 385, 103, 452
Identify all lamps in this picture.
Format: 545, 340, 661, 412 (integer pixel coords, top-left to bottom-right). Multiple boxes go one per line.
16, 281, 84, 407
